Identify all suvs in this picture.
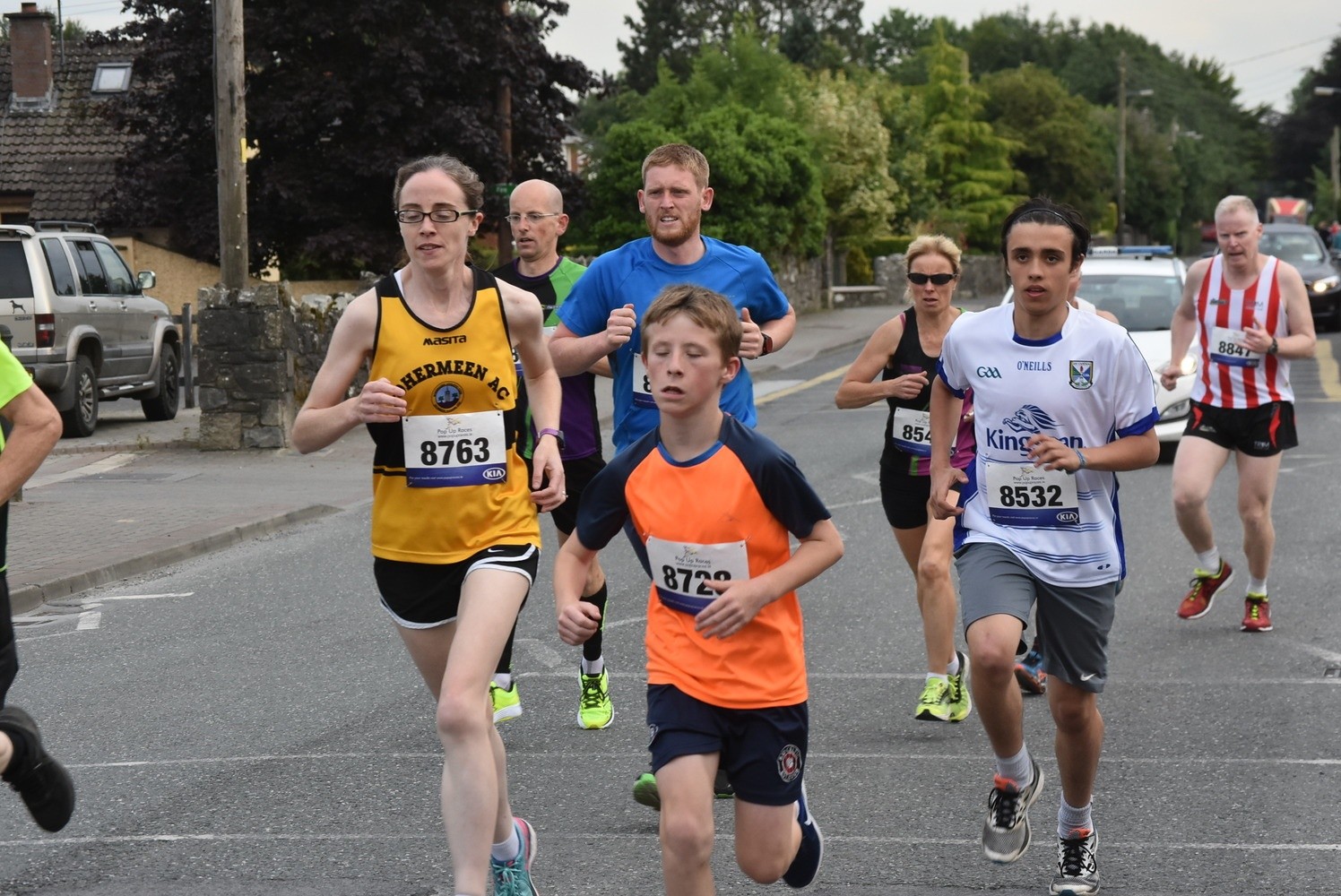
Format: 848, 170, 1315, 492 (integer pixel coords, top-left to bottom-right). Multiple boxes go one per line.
0, 220, 183, 439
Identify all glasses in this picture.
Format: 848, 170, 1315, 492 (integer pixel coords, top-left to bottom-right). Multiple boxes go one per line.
505, 213, 561, 225
394, 209, 480, 223
905, 271, 958, 286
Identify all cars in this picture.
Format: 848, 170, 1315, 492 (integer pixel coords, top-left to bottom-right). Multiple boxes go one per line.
999, 244, 1205, 457
1198, 221, 1341, 332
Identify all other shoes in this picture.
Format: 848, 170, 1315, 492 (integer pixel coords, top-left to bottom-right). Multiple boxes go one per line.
714, 770, 734, 797
633, 772, 661, 809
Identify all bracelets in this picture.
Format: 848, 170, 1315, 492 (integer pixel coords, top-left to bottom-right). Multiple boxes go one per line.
1068, 447, 1087, 474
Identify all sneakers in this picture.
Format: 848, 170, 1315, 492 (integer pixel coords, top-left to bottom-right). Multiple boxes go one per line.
915, 675, 950, 722
947, 651, 972, 722
490, 818, 538, 896
1013, 651, 1046, 693
980, 754, 1047, 865
577, 663, 615, 731
783, 779, 825, 889
1240, 594, 1273, 631
1047, 826, 1100, 895
1178, 559, 1237, 620
487, 681, 522, 721
0, 706, 73, 834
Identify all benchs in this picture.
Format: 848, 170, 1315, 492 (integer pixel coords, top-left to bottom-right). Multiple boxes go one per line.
827, 284, 888, 308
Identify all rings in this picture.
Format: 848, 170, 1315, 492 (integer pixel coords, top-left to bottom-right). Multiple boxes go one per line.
562, 490, 569, 499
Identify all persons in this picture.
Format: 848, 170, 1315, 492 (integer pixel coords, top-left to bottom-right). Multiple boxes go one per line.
554, 283, 844, 896
547, 143, 797, 808
833, 231, 979, 723
291, 152, 566, 896
1012, 265, 1119, 695
1162, 193, 1317, 631
927, 201, 1161, 895
481, 180, 615, 729
0, 330, 75, 833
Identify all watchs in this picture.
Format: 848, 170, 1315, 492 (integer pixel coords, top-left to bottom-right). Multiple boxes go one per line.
1266, 338, 1278, 357
535, 428, 566, 452
760, 332, 773, 358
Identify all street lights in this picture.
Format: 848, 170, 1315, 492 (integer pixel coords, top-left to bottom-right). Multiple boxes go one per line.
1117, 66, 1155, 242
